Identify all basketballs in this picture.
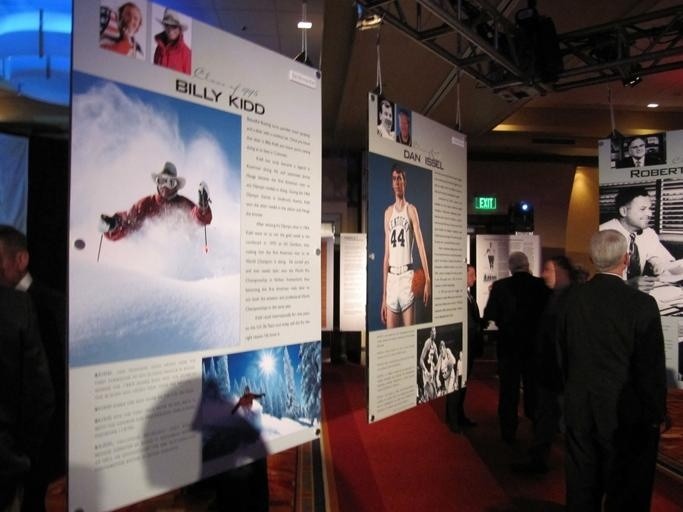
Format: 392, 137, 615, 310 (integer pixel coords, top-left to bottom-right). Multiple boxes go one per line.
412, 268, 427, 299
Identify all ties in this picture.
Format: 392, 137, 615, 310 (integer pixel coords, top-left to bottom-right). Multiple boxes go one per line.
625, 233, 640, 280
635, 159, 641, 167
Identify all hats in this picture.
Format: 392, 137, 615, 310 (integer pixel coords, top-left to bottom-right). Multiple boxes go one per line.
151, 161, 186, 190
154, 7, 189, 32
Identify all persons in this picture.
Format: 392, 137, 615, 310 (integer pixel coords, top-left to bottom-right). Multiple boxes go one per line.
482, 250, 548, 447
98, 159, 212, 241
378, 167, 432, 330
614, 137, 661, 166
397, 106, 411, 145
0, 283, 56, 510
453, 350, 463, 389
544, 228, 670, 512
435, 341, 457, 394
530, 255, 579, 476
376, 97, 394, 138
0, 225, 67, 511
230, 384, 266, 424
596, 186, 682, 295
99, 3, 143, 59
151, 8, 190, 74
443, 263, 486, 434
418, 328, 439, 400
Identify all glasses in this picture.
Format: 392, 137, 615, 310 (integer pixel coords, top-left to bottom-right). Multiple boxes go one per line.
155, 177, 179, 190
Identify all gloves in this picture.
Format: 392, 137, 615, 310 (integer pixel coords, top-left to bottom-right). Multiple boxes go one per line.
101, 214, 118, 232
199, 185, 209, 207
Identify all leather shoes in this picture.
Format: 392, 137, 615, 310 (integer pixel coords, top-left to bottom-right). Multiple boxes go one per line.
450, 421, 460, 432
458, 416, 477, 426
